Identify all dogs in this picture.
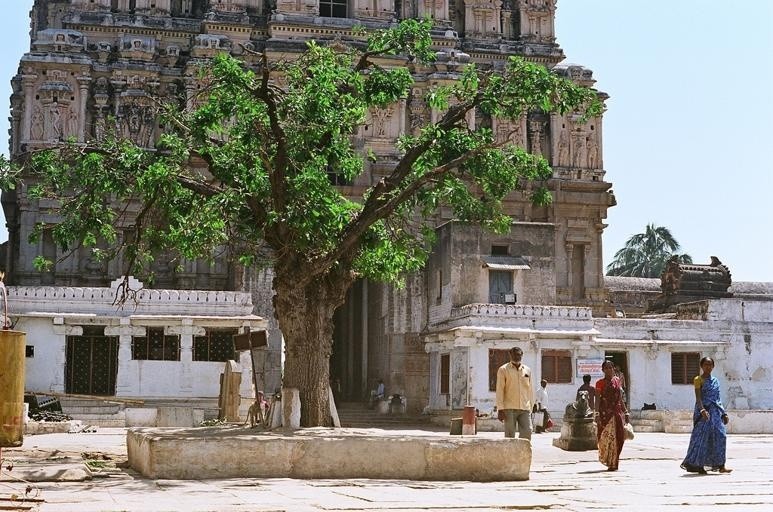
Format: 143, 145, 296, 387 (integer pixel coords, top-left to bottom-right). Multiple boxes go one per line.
565, 390, 594, 417
243, 390, 265, 428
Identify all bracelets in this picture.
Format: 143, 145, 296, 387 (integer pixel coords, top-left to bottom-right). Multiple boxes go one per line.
699, 409, 706, 413
624, 410, 629, 415
595, 412, 600, 416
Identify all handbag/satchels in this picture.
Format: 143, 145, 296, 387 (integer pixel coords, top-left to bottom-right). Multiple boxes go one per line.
533, 409, 553, 428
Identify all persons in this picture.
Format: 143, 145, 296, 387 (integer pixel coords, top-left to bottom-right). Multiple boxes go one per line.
595, 360, 629, 471
586, 133, 598, 168
614, 366, 626, 388
255, 391, 270, 422
369, 378, 384, 410
558, 130, 570, 165
577, 374, 596, 417
535, 380, 548, 434
680, 356, 733, 474
332, 378, 343, 409
496, 348, 534, 441
